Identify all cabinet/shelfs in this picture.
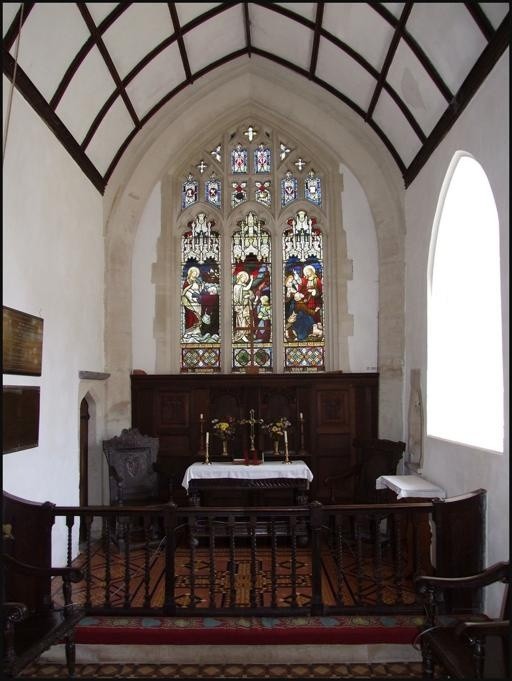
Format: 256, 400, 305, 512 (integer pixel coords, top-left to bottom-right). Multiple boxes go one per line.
131, 374, 379, 478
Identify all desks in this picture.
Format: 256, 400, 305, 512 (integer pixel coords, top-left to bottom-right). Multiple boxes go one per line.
182, 461, 314, 547
375, 475, 446, 577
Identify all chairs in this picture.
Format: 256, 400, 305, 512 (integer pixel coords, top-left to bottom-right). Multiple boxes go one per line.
101, 427, 176, 552
0, 552, 85, 680
323, 436, 406, 555
412, 561, 511, 680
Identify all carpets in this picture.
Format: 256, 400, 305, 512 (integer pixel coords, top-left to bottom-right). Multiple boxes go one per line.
19, 662, 455, 681
53, 538, 489, 646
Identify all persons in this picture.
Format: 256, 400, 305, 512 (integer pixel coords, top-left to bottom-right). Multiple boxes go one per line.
181, 264, 323, 341
